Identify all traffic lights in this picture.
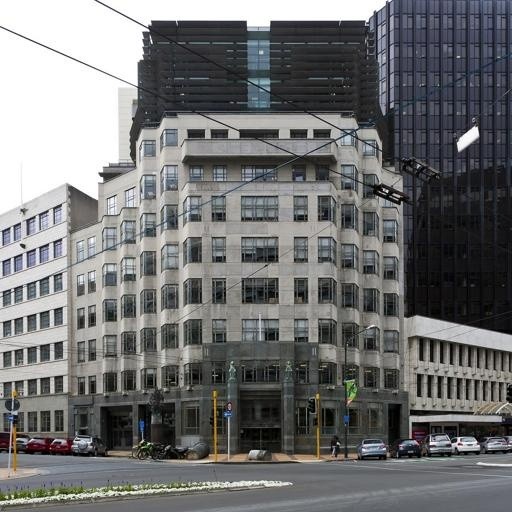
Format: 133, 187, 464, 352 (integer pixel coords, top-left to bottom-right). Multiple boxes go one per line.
309, 397, 317, 414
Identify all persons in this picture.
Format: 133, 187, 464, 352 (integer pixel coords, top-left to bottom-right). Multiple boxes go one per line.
330, 435, 341, 453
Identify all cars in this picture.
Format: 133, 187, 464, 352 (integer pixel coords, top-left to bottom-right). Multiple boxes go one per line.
1, 429, 70, 457
355, 433, 511, 464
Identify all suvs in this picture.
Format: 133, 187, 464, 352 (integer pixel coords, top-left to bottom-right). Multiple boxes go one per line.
71, 434, 108, 459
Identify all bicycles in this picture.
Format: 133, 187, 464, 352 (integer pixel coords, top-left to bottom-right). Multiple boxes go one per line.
131, 436, 163, 461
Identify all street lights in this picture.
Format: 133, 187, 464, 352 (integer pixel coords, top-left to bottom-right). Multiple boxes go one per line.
343, 324, 379, 459
